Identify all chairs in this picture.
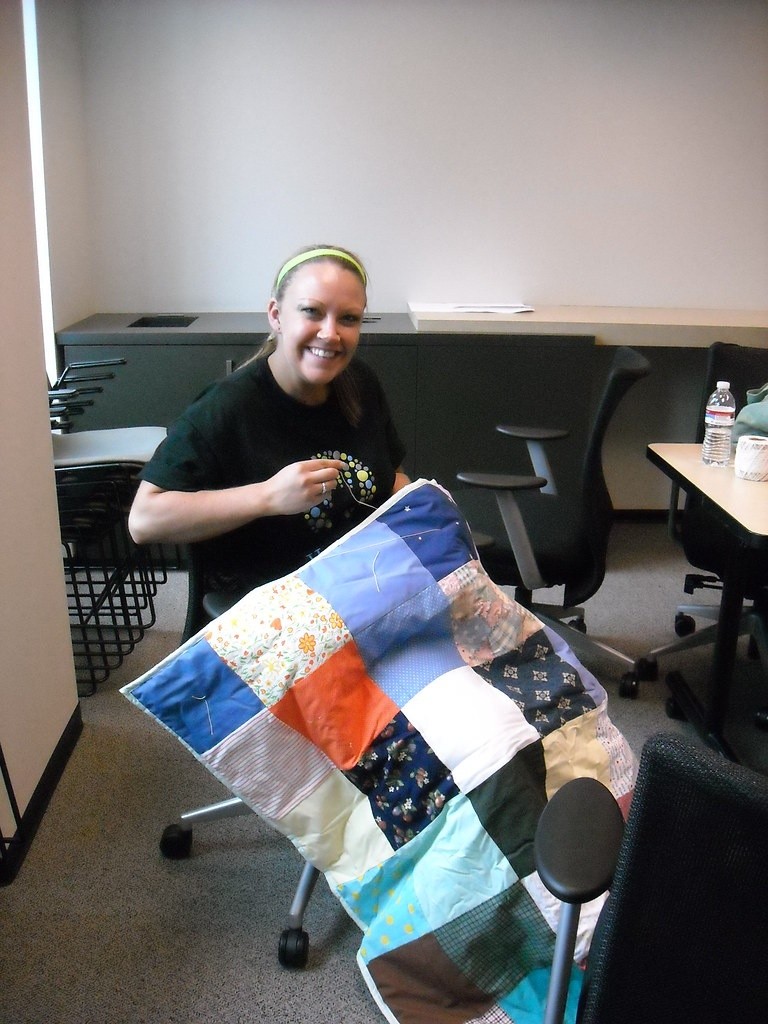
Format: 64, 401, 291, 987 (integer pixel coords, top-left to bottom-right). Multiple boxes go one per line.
634, 341, 768, 733
533, 732, 767, 1024
161, 378, 320, 968
449, 346, 652, 707
47, 358, 168, 696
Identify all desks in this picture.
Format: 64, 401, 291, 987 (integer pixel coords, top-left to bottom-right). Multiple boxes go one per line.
645, 442, 768, 764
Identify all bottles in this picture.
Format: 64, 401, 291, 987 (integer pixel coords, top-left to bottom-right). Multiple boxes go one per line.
702, 381, 736, 468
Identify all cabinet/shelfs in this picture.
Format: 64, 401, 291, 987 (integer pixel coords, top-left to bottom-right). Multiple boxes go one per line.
54, 312, 597, 572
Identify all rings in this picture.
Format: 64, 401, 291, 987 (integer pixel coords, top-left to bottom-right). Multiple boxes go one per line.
322, 483, 327, 493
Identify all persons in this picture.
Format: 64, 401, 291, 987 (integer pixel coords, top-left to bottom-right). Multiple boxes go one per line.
127, 245, 414, 631
